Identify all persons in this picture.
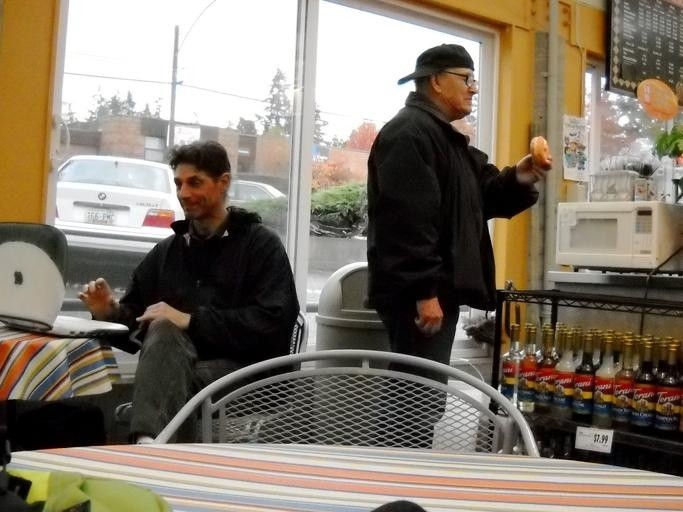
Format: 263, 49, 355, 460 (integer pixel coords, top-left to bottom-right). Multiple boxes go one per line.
675, 150, 683, 165
565, 142, 588, 170
366, 44, 553, 449
76, 141, 300, 445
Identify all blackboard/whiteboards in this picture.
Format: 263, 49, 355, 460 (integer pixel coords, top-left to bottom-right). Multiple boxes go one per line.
609, 0, 683, 93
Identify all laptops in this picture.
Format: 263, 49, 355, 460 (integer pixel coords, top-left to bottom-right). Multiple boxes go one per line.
0, 240, 129, 339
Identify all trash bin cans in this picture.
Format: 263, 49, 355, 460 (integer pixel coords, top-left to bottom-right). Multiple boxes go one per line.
314, 263, 392, 445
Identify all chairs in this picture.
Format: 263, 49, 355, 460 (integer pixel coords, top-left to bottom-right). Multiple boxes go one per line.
194, 309, 307, 444
149, 347, 542, 459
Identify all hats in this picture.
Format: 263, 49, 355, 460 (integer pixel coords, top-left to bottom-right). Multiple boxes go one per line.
396, 42, 475, 86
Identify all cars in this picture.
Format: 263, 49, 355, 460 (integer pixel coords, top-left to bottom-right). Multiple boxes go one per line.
53, 154, 288, 276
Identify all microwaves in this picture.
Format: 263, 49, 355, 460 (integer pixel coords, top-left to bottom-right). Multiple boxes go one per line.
554, 201, 682, 274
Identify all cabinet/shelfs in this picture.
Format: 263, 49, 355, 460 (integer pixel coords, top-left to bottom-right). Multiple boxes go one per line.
489, 289, 683, 476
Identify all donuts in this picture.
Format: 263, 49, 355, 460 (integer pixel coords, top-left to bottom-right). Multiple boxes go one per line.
530, 137, 550, 168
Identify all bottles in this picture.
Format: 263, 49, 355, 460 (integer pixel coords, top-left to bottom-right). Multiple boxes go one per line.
497, 321, 682, 457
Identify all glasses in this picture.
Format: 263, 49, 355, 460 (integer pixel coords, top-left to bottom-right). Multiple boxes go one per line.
443, 68, 479, 92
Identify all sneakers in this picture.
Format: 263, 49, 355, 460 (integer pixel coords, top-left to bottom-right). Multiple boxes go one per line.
114, 400, 133, 425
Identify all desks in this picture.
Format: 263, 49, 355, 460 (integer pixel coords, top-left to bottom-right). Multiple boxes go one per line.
0, 319, 122, 453
0, 441, 683, 512
